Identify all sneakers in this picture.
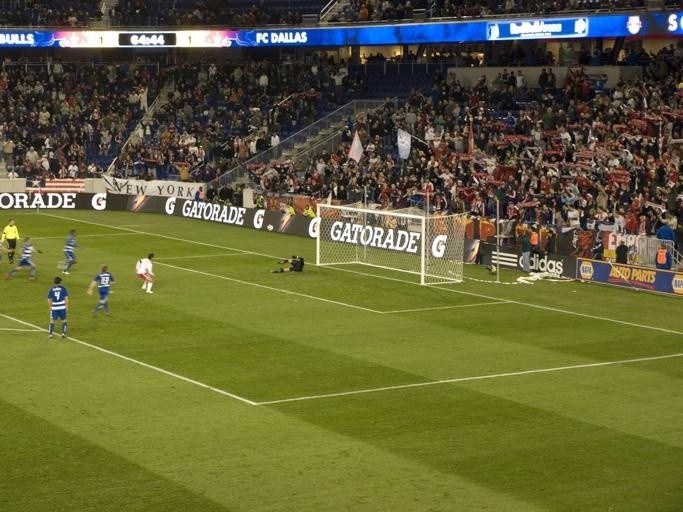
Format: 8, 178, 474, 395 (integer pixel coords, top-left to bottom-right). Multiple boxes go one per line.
145, 290, 153, 294
9, 260, 13, 264
62, 271, 70, 274
48, 335, 52, 338
141, 286, 146, 290
61, 333, 65, 338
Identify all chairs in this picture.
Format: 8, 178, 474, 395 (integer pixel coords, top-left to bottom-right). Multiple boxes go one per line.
86, 60, 434, 182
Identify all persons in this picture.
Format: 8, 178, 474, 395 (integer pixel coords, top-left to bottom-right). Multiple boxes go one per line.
46, 277, 68, 338
0, 1, 683, 277
3, 237, 42, 281
86, 265, 116, 317
134, 253, 155, 294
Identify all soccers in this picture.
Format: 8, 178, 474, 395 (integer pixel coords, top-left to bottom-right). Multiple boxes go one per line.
267, 225, 272, 230
